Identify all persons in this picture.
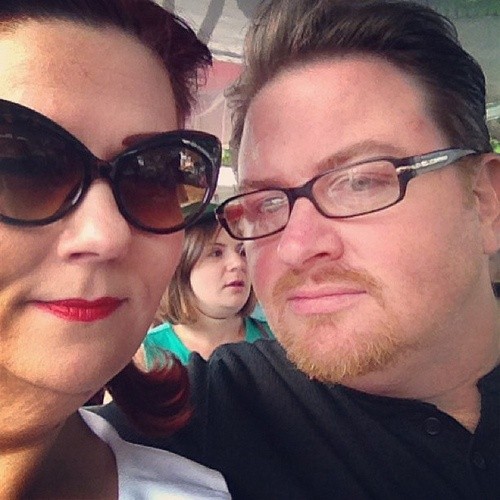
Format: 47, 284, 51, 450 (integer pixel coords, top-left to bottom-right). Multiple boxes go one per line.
97, 0, 500, 500
131, 201, 277, 373
0, 0, 233, 500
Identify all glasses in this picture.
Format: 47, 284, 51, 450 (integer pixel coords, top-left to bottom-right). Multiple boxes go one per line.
0, 99, 222, 235
214, 147, 480, 242
